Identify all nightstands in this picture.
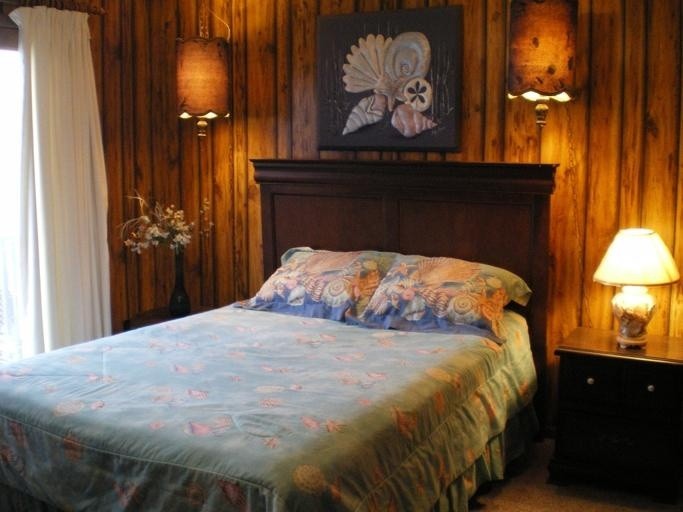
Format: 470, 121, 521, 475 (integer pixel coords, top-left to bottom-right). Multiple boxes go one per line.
123, 302, 221, 331
546, 327, 681, 505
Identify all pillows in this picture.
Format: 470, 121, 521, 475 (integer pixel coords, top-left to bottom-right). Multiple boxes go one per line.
358, 255, 532, 345
234, 246, 400, 324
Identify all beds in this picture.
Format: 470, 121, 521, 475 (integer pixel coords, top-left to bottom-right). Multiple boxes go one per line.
0, 160, 560, 510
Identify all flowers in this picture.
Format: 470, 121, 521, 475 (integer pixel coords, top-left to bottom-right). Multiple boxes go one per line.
114, 189, 213, 256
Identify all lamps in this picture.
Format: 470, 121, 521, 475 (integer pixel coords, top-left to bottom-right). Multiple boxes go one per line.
593, 227, 682, 350
176, 0, 233, 138
506, 0, 576, 129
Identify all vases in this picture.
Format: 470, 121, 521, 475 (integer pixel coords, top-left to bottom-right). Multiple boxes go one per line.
169, 245, 190, 320
